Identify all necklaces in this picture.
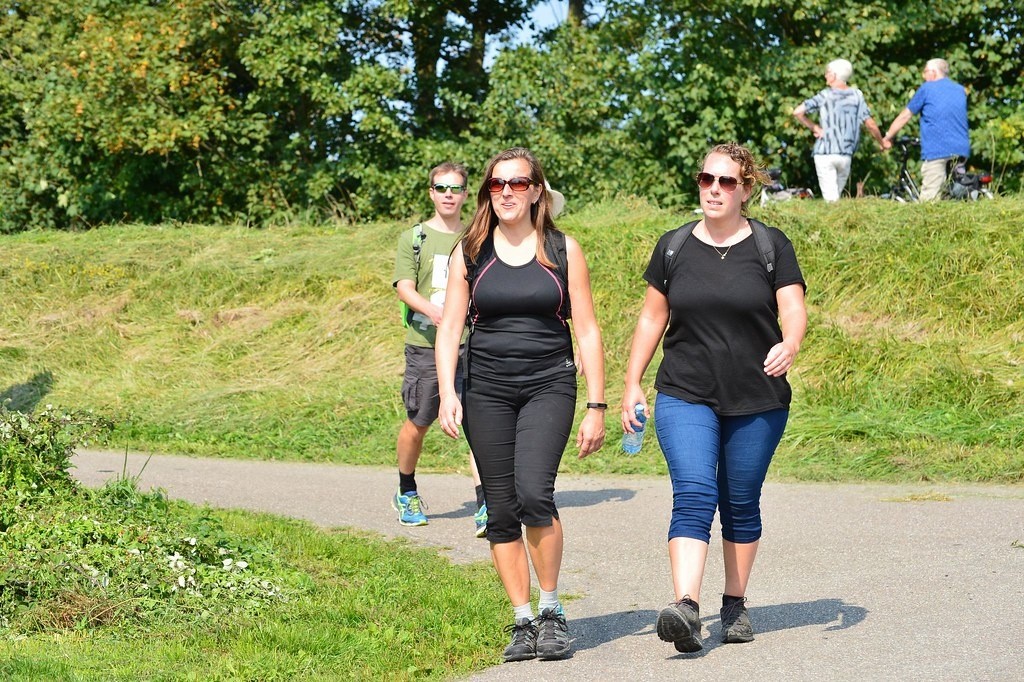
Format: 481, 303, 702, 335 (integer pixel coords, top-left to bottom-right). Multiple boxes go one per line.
702, 221, 743, 260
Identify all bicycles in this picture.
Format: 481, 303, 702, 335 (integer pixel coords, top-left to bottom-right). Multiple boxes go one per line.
757, 169, 813, 203
880, 139, 994, 203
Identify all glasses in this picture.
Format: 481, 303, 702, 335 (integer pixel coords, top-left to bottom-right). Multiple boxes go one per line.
486, 177, 538, 191
696, 173, 741, 191
432, 183, 464, 194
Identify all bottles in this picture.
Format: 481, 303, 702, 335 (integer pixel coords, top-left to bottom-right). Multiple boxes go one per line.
622, 404, 646, 453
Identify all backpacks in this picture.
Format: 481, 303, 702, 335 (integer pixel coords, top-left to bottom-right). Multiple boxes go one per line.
398, 223, 426, 329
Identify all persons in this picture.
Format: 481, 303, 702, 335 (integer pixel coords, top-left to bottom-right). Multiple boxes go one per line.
620, 144, 807, 653
881, 58, 969, 203
390, 162, 491, 538
434, 147, 605, 662
793, 59, 890, 202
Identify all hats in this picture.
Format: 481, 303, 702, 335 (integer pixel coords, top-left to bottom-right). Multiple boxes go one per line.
544, 179, 564, 218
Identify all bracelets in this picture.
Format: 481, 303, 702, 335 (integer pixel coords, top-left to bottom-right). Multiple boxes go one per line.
587, 403, 607, 410
885, 132, 893, 142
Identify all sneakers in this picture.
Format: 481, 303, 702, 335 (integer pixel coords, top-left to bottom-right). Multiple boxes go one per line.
391, 485, 429, 526
536, 607, 570, 658
720, 597, 754, 643
503, 617, 539, 660
656, 593, 703, 652
474, 500, 488, 537
